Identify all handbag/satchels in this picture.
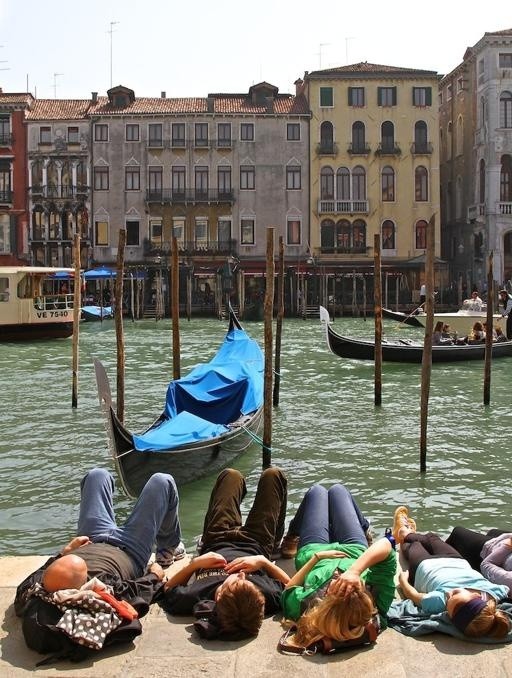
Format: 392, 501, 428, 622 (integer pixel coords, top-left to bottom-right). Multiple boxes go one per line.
279, 615, 380, 656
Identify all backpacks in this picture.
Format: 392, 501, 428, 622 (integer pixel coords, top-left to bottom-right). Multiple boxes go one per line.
20, 596, 148, 667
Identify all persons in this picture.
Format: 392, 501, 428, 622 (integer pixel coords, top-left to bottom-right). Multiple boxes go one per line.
442, 324, 450, 337
432, 321, 451, 346
392, 507, 508, 637
470, 291, 482, 311
495, 327, 507, 343
444, 527, 512, 601
13, 468, 184, 619
280, 484, 397, 648
469, 322, 484, 344
497, 290, 512, 341
482, 322, 497, 339
505, 280, 512, 295
162, 469, 286, 640
420, 284, 426, 305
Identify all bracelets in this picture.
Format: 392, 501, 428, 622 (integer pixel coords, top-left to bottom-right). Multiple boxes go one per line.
314, 553, 321, 560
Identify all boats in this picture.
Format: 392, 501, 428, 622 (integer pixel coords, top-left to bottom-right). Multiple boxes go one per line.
78, 304, 115, 320
382, 307, 425, 328
382, 308, 423, 327
409, 302, 508, 339
413, 312, 509, 335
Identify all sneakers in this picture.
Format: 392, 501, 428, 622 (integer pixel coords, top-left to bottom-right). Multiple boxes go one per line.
393, 507, 408, 546
193, 534, 204, 556
156, 543, 186, 566
409, 518, 418, 533
281, 538, 299, 558
